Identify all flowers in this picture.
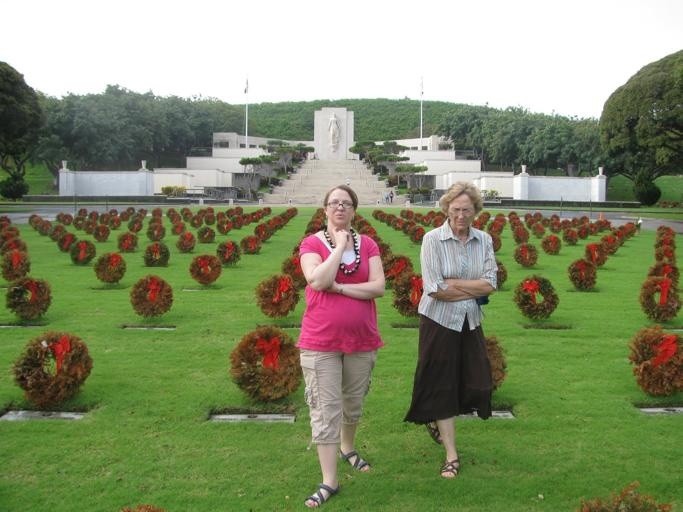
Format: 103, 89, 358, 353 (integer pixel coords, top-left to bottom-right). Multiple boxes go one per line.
1, 207, 683, 409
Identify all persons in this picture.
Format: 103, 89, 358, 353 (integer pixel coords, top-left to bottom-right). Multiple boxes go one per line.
398, 180, 501, 480
382, 192, 388, 203
291, 184, 387, 509
389, 191, 393, 203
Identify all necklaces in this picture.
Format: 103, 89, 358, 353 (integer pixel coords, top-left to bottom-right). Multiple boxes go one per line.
322, 226, 362, 275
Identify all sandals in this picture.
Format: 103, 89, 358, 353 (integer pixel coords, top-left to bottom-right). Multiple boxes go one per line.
440, 457, 459, 478
340, 448, 370, 472
427, 422, 443, 444
305, 483, 339, 506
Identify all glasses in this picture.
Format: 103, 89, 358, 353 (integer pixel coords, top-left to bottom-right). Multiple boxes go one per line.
326, 201, 354, 210
451, 210, 474, 217
